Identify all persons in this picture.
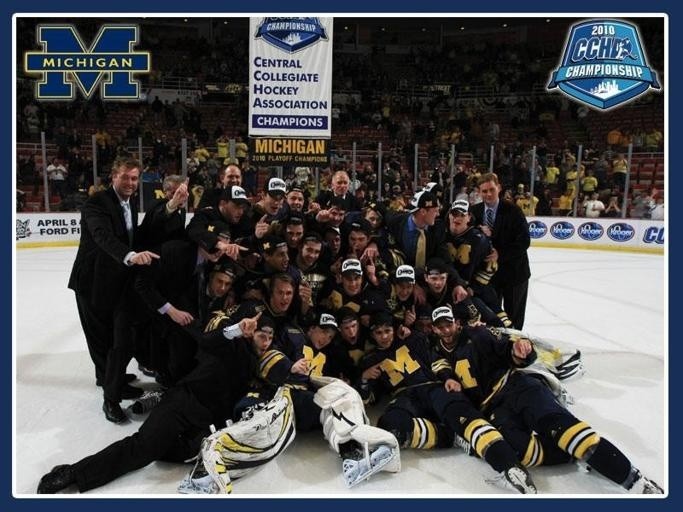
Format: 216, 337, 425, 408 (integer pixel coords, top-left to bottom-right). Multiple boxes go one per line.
17, 13, 667, 495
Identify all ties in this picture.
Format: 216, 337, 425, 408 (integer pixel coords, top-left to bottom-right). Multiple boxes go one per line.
124, 204, 133, 248
486, 209, 494, 230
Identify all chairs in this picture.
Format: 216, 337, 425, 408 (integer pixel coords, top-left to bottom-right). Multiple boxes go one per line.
539, 95, 664, 147
71, 101, 149, 159
18, 140, 69, 211
194, 99, 247, 142
330, 114, 432, 168
612, 153, 664, 219
471, 105, 539, 162
133, 109, 193, 151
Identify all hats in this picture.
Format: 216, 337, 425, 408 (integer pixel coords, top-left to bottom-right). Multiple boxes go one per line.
347, 218, 373, 234
263, 177, 288, 195
430, 306, 453, 322
256, 230, 288, 251
415, 182, 443, 192
211, 262, 236, 278
313, 313, 338, 329
340, 258, 363, 275
370, 202, 388, 221
404, 191, 439, 214
394, 265, 414, 280
219, 185, 251, 208
451, 199, 471, 215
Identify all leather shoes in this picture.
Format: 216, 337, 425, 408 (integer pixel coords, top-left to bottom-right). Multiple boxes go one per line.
103, 400, 128, 424
119, 386, 145, 400
37, 465, 71, 493
96, 374, 137, 385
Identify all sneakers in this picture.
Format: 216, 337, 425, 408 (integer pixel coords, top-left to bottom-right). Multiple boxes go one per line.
451, 433, 475, 457
504, 463, 535, 494
622, 465, 663, 495
338, 438, 395, 489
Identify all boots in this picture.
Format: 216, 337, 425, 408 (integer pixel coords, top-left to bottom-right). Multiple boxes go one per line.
133, 388, 164, 414
